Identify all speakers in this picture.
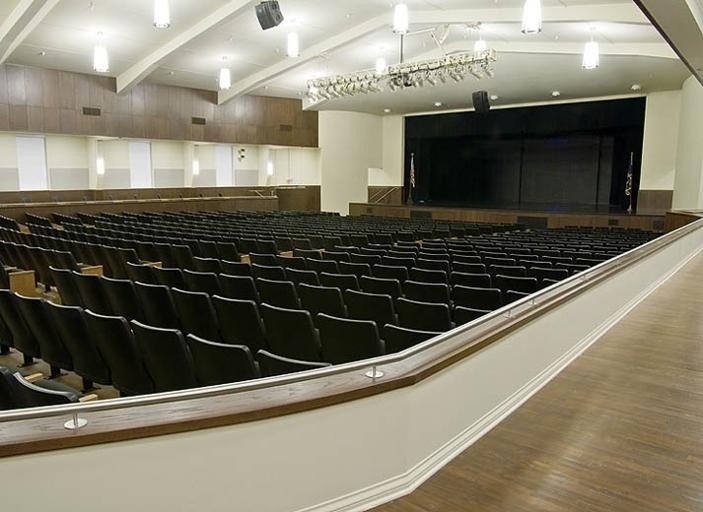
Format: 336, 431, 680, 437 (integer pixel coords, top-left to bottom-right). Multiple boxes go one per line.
254, 0, 285, 30
472, 91, 490, 115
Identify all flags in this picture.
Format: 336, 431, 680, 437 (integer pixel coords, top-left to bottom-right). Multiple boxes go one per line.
410, 157, 416, 188
625, 164, 632, 196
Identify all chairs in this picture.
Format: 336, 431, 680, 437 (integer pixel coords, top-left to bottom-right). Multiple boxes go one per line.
0, 207, 668, 405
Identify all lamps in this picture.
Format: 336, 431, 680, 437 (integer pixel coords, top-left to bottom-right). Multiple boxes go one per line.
218, 56, 231, 91
286, 22, 299, 57
299, 36, 497, 109
93, 32, 110, 72
520, 0, 540, 36
155, 4, 170, 30
391, 1, 410, 34
581, 27, 599, 69
